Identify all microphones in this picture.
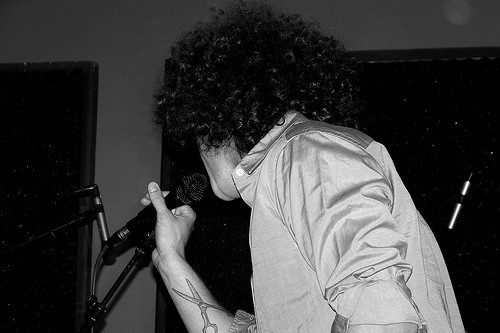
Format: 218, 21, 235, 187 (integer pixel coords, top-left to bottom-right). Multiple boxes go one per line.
447, 163, 483, 230
105, 173, 208, 249
90, 184, 115, 265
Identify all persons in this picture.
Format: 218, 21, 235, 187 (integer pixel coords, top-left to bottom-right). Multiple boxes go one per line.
139, 0, 466, 333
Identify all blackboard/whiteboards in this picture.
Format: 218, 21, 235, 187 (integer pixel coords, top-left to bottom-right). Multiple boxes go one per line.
155, 47, 500, 333
0, 62, 99, 333
